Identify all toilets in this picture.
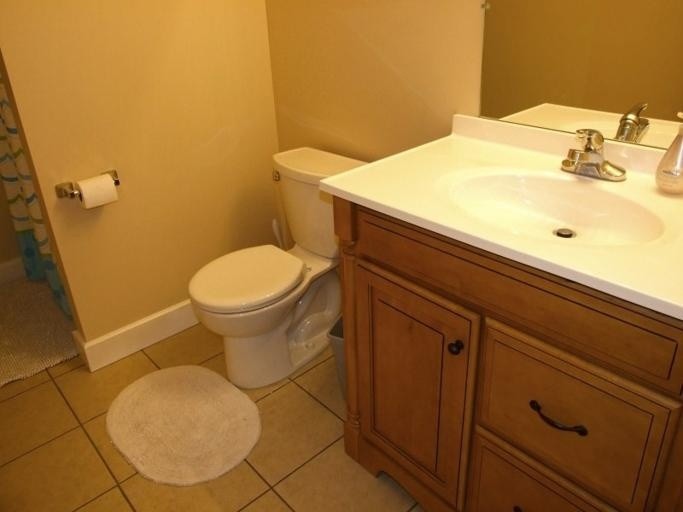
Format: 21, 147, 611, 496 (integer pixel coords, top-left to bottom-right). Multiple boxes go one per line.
187, 146, 371, 390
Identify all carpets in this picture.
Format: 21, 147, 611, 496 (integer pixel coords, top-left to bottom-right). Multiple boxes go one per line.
0, 276, 76, 387
103, 363, 261, 486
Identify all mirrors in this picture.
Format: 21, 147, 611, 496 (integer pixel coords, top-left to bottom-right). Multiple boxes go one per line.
476, 1, 682, 152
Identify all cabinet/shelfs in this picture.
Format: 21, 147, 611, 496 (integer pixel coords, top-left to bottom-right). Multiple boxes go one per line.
335, 206, 683, 512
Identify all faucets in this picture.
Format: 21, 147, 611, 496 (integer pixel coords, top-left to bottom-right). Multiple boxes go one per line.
612, 101, 650, 144
561, 128, 628, 181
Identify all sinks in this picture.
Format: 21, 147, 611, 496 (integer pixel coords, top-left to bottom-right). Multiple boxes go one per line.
560, 118, 677, 150
433, 167, 666, 251
